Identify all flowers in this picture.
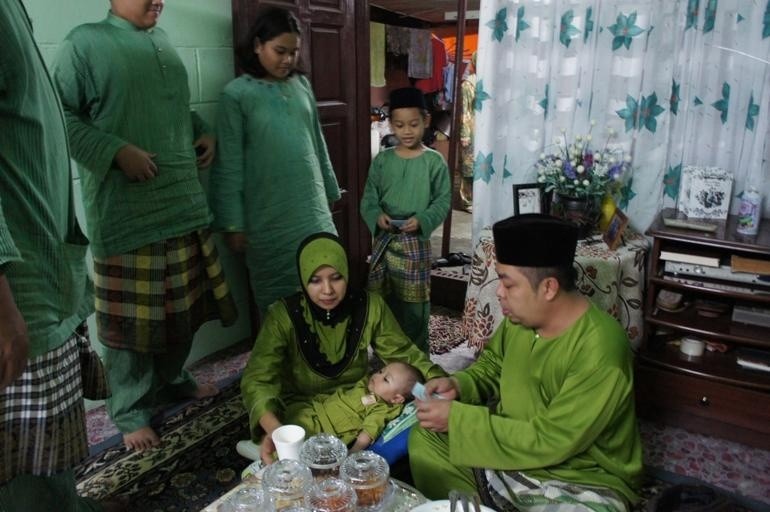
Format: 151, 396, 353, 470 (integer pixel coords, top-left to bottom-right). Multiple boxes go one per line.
536, 118, 632, 197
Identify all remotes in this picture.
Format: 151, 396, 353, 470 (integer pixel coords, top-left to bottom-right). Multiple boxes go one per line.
664, 217, 718, 232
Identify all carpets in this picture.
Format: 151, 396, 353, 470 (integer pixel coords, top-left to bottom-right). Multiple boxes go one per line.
67, 369, 769, 511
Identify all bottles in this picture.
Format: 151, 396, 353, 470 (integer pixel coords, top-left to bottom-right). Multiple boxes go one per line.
735, 185, 764, 235
222, 433, 390, 512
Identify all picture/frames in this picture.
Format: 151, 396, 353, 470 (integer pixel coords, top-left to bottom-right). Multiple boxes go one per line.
601, 207, 630, 251
512, 182, 548, 219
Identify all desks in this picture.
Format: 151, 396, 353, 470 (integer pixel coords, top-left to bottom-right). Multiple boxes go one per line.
186, 457, 430, 512
478, 222, 642, 357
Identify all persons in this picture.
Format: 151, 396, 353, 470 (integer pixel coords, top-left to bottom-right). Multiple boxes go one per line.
405, 213, 645, 511
357, 88, 454, 361
50, 0, 238, 455
240, 230, 451, 467
209, 7, 342, 330
0, 0, 97, 508
235, 359, 428, 461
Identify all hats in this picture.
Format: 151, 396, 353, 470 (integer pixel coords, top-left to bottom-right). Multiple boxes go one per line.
389, 88, 425, 109
494, 214, 578, 267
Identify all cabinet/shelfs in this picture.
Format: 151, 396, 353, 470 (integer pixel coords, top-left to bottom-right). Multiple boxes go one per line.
631, 206, 768, 452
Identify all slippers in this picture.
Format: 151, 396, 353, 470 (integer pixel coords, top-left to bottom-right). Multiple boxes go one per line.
435, 252, 471, 266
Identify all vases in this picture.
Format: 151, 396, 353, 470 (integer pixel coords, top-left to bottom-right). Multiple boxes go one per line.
554, 199, 596, 241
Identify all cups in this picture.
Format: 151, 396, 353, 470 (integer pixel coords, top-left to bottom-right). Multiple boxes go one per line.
271, 425, 306, 461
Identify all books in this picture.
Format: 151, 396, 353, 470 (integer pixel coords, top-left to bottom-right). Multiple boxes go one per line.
736, 349, 770, 374
659, 245, 723, 267
730, 250, 769, 278
